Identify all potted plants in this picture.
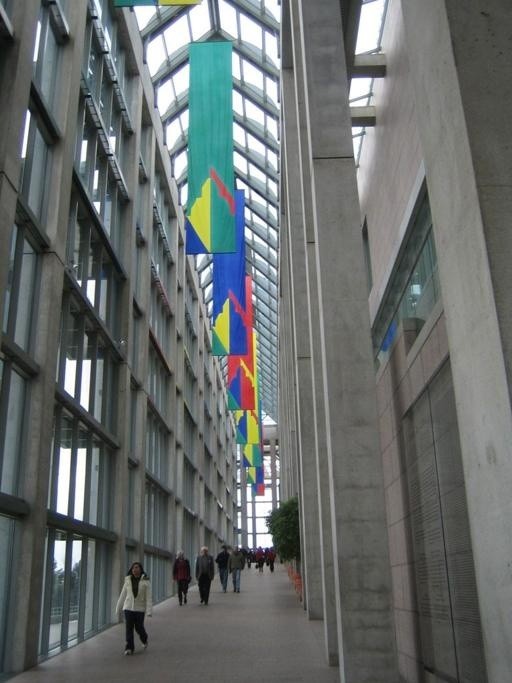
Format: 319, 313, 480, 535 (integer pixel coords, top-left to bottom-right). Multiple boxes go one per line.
265, 495, 302, 604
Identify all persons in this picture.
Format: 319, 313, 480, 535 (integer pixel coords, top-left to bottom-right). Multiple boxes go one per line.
244, 546, 278, 572
194, 545, 215, 605
116, 563, 149, 657
229, 547, 244, 594
169, 549, 192, 604
216, 545, 230, 592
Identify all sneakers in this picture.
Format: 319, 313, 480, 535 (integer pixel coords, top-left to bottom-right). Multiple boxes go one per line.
123, 643, 148, 656
